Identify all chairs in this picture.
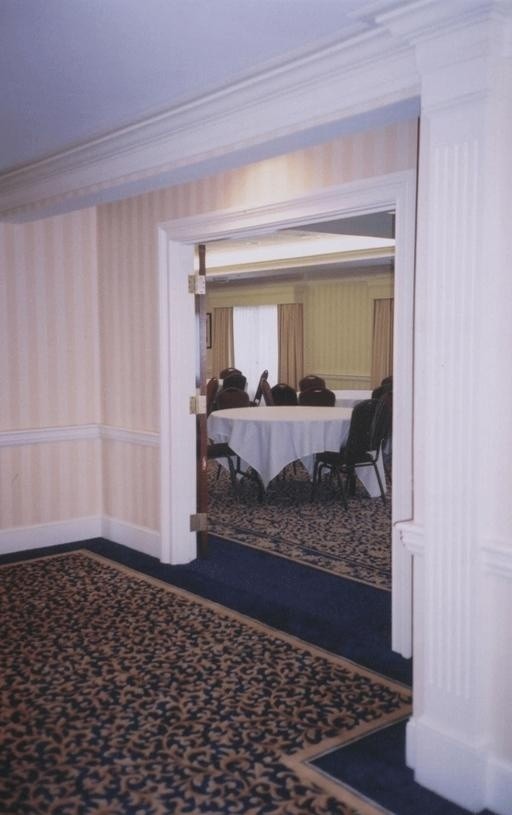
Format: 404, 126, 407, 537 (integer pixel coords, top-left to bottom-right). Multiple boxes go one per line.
206, 367, 335, 486
309, 375, 392, 512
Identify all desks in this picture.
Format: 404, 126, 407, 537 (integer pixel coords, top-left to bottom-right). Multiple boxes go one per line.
210, 405, 356, 504
